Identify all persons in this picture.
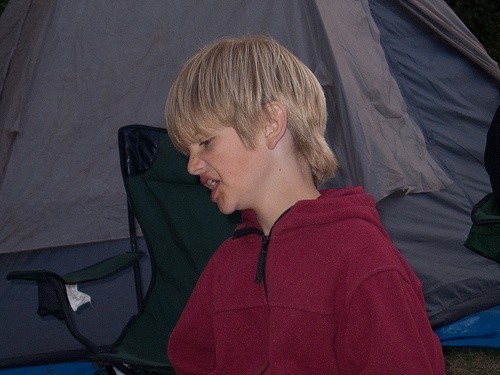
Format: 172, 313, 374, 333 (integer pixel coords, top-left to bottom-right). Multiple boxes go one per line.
165, 34, 446, 375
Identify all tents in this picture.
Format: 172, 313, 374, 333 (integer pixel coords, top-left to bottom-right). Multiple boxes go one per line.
0, 0, 500, 367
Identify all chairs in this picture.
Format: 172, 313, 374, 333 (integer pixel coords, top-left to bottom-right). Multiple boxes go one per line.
6, 124, 240, 375
